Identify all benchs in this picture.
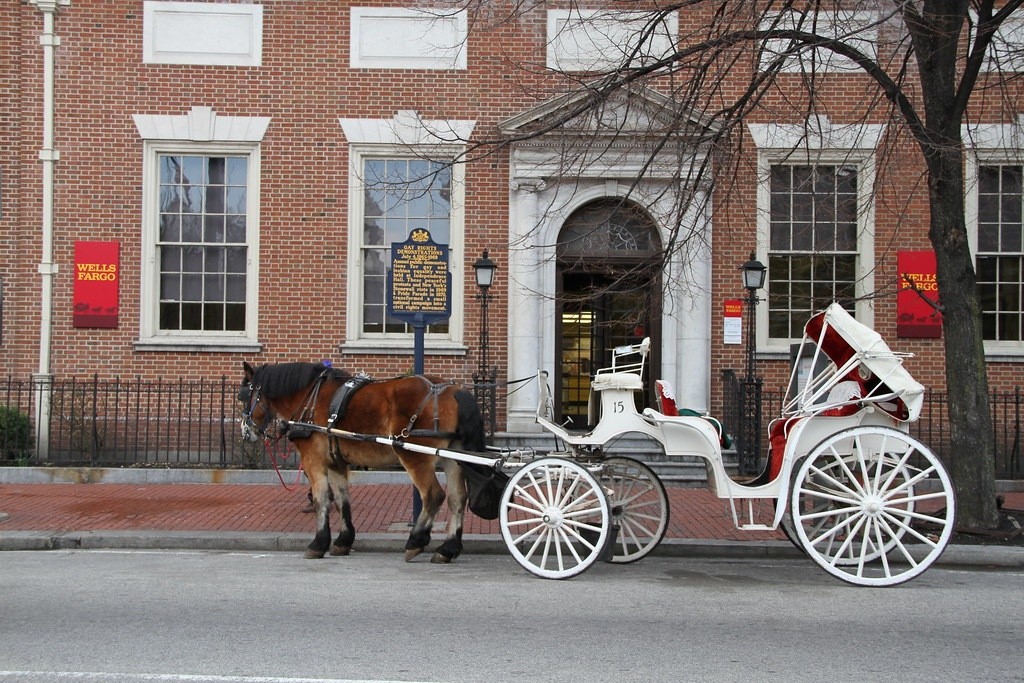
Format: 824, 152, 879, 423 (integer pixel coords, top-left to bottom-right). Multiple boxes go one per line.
592, 337, 650, 391
653, 378, 731, 449
771, 381, 861, 449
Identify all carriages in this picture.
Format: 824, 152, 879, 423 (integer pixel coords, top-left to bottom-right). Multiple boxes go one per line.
236, 302, 956, 588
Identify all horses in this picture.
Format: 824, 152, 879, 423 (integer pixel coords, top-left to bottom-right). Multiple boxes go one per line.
241, 361, 484, 564
